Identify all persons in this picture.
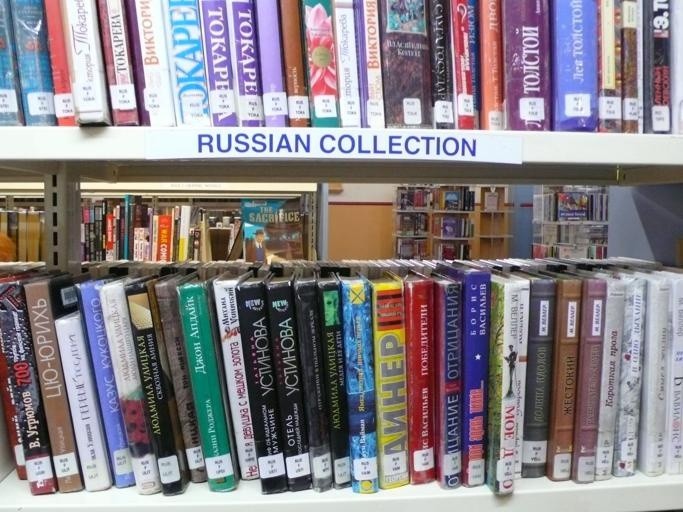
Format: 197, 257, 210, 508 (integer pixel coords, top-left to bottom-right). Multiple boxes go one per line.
247, 230, 266, 262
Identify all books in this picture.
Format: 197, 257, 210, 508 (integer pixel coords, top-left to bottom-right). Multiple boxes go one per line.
533, 243, 555, 259
245, 200, 305, 264
431, 215, 474, 237
533, 224, 557, 245
558, 226, 578, 243
81, 195, 243, 262
396, 213, 430, 235
556, 191, 588, 221
396, 238, 429, 258
439, 190, 475, 211
589, 245, 608, 259
532, 193, 556, 221
433, 240, 471, 259
555, 245, 588, 259
484, 192, 498, 211
575, 225, 608, 245
1, 0, 670, 133
588, 191, 608, 222
1, 257, 683, 496
0, 205, 45, 262
396, 186, 439, 210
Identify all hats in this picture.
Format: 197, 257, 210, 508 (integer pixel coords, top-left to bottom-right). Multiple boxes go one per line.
253, 229, 263, 234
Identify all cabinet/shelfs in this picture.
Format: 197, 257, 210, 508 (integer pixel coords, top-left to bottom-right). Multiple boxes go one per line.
0, 123, 683, 511
530, 185, 610, 260
392, 185, 514, 261
